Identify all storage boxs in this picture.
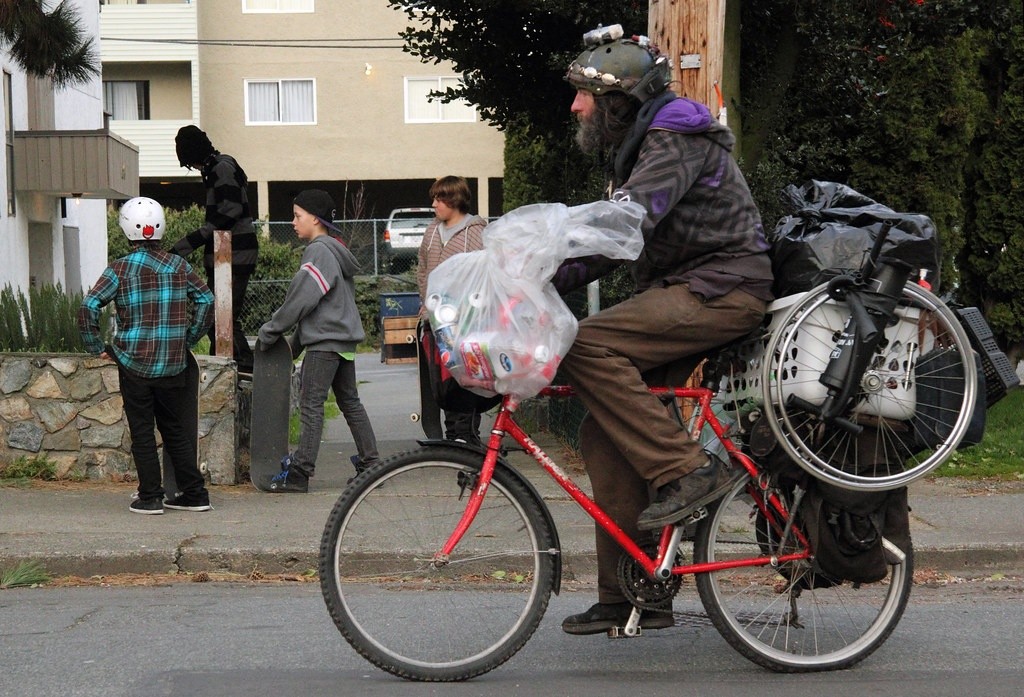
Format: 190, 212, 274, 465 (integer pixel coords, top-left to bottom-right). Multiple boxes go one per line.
715, 290, 939, 421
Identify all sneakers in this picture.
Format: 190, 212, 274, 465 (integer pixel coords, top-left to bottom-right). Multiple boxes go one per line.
260, 454, 309, 492
130, 496, 164, 514
348, 453, 384, 489
162, 493, 210, 511
561, 599, 674, 634
638, 449, 734, 530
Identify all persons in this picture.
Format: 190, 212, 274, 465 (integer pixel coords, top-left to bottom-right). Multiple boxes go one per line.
549, 22, 777, 638
257, 188, 387, 495
77, 196, 218, 515
165, 124, 260, 378
415, 174, 488, 445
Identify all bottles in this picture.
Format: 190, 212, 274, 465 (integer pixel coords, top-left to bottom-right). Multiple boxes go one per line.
430, 279, 576, 414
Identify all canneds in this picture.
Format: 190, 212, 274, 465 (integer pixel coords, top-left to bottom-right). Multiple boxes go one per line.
427, 292, 489, 371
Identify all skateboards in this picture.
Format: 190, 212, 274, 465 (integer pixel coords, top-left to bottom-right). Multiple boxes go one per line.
406, 316, 445, 442
249, 332, 296, 494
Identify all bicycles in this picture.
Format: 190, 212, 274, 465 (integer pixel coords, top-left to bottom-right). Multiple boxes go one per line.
313, 231, 915, 680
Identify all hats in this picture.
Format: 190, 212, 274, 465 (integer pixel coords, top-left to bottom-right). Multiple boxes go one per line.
175, 125, 212, 164
294, 190, 343, 233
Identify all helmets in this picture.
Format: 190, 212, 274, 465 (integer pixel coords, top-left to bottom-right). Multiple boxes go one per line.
564, 23, 671, 104
120, 196, 166, 240
